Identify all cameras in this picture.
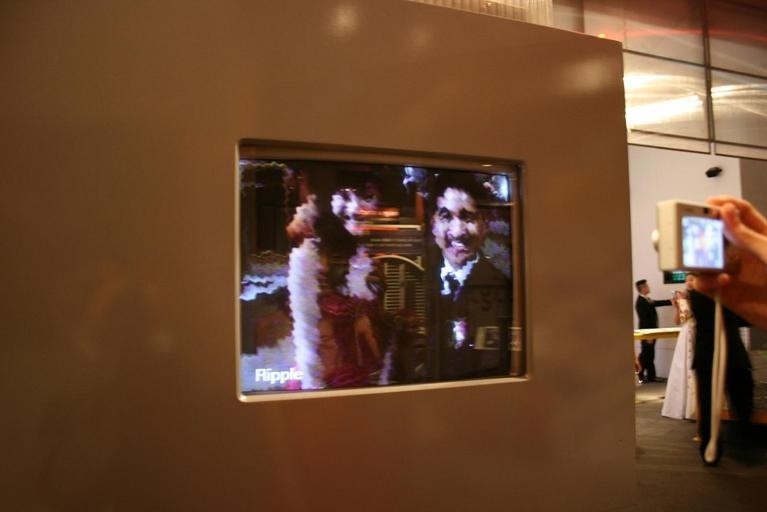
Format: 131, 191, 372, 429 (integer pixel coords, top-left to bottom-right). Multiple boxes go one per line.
651, 201, 741, 275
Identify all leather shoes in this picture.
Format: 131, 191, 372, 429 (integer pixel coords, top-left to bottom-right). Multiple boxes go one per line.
638, 368, 666, 383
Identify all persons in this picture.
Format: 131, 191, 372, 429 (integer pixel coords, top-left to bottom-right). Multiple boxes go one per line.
679, 193, 766, 336
663, 269, 701, 421
417, 169, 511, 383
281, 158, 405, 393
683, 284, 760, 466
635, 279, 674, 384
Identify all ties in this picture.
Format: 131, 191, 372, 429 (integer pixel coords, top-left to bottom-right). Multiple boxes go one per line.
445, 275, 459, 297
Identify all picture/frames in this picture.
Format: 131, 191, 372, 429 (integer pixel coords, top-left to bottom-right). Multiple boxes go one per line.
235, 138, 531, 402
